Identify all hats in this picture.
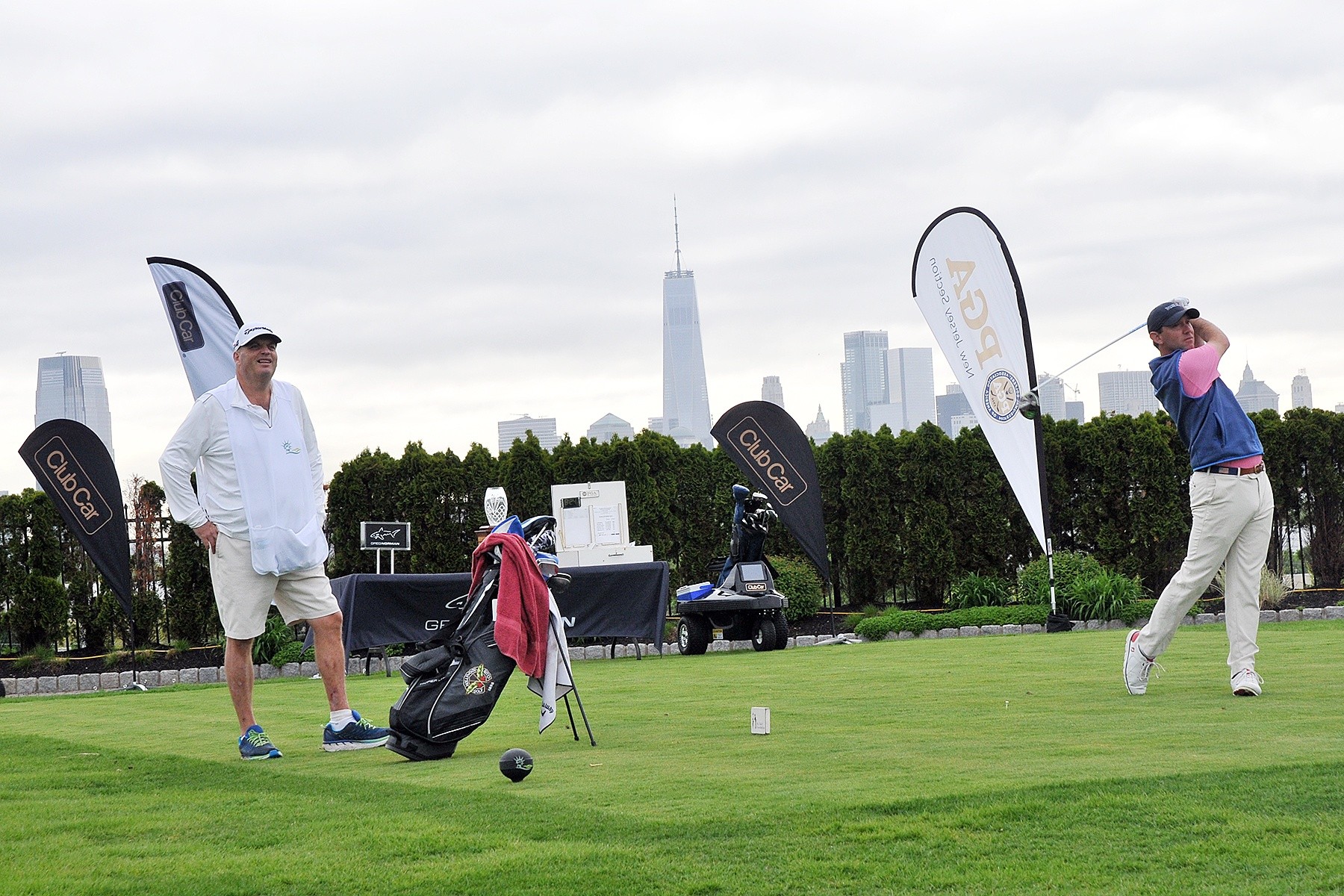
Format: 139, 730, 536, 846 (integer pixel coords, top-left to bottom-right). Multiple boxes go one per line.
1147, 302, 1200, 334
233, 322, 282, 352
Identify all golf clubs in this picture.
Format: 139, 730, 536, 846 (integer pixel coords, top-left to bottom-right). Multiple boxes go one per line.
526, 529, 573, 595
741, 491, 778, 535
1017, 297, 1191, 421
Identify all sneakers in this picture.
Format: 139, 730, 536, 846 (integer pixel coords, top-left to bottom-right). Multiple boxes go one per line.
1231, 668, 1264, 697
320, 710, 389, 752
1122, 629, 1166, 695
238, 725, 283, 760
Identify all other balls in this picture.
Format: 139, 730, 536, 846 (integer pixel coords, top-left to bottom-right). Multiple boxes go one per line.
499, 748, 533, 779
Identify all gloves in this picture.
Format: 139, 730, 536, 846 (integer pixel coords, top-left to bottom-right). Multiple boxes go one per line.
1172, 298, 1190, 306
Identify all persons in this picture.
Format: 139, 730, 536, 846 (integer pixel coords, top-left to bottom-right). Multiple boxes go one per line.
158, 323, 390, 760
1123, 298, 1275, 696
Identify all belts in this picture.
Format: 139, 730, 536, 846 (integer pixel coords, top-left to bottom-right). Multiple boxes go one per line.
1195, 461, 1264, 476
